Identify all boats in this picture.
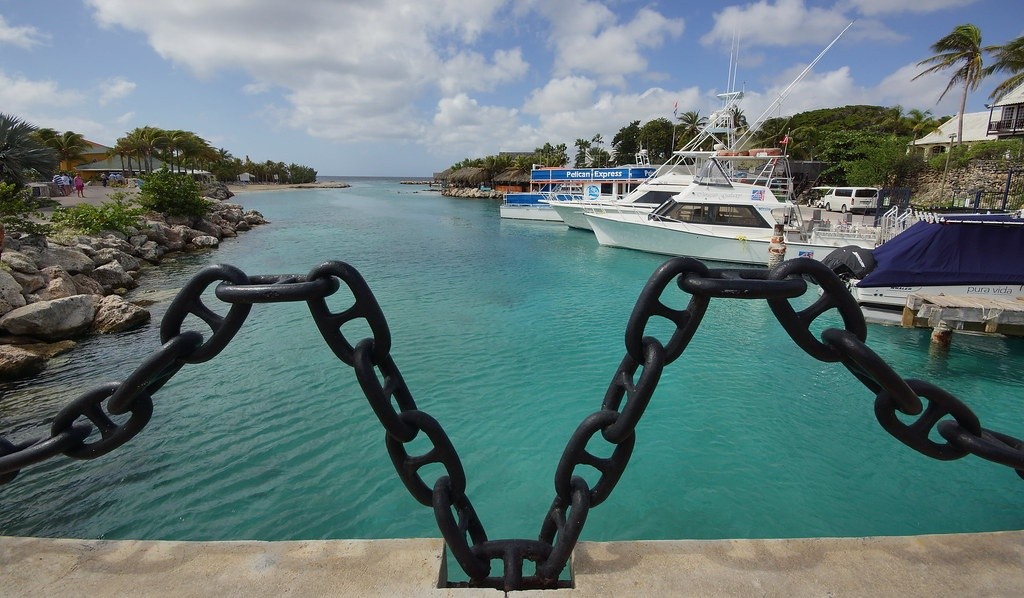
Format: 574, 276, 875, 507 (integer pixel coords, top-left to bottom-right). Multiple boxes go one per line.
815, 210, 1024, 339
499, 149, 837, 221
580, 155, 875, 271
536, 28, 822, 234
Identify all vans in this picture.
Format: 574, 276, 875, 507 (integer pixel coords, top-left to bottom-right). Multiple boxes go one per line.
823, 186, 880, 214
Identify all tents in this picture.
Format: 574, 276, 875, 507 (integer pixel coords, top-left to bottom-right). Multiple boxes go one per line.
73, 153, 211, 174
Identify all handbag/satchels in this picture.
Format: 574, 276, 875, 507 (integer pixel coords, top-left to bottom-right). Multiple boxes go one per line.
82, 183, 84, 187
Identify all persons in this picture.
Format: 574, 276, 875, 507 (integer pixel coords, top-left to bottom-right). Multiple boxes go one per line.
74, 173, 84, 198
52, 173, 75, 197
101, 172, 124, 187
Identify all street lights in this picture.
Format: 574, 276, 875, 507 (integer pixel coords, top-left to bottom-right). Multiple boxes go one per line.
937, 133, 958, 209
